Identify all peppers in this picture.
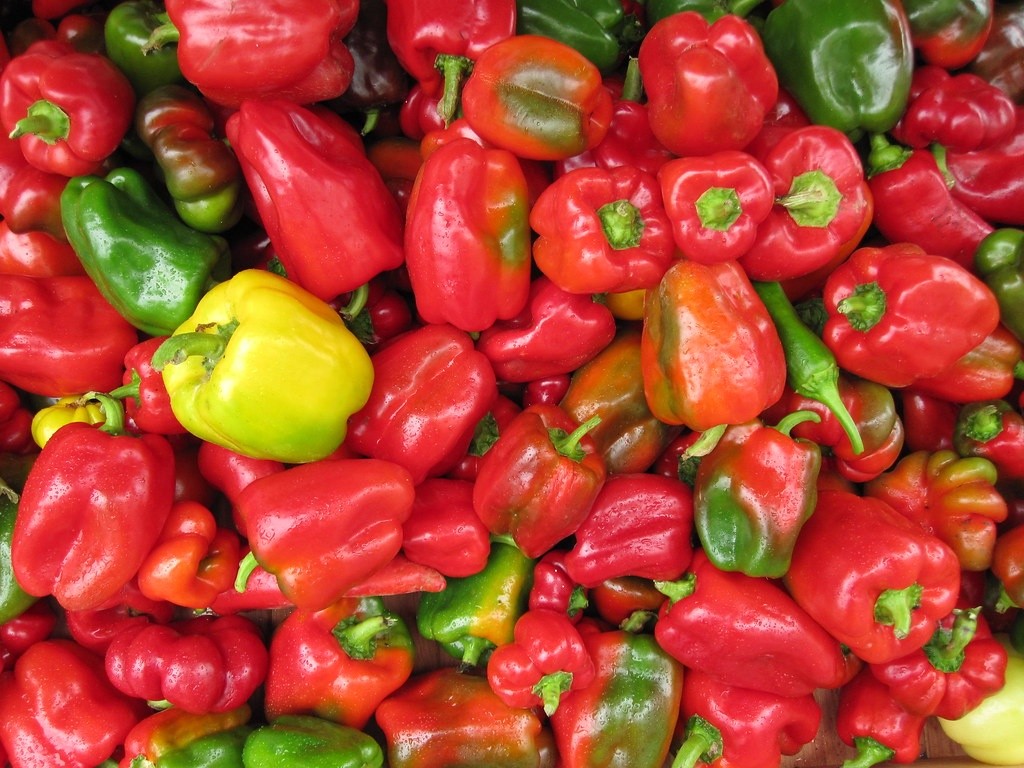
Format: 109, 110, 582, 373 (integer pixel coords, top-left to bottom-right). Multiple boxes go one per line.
0, 0, 1024, 768
